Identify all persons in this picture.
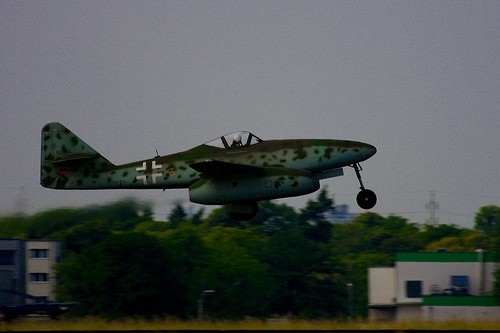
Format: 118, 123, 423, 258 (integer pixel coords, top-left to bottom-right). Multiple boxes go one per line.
230, 135, 242, 147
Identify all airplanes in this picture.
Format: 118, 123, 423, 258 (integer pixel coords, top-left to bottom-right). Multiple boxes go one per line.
0, 289, 79, 320
40, 122, 377, 221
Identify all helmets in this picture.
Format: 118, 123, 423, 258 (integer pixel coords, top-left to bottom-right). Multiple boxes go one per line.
232, 134, 242, 143
